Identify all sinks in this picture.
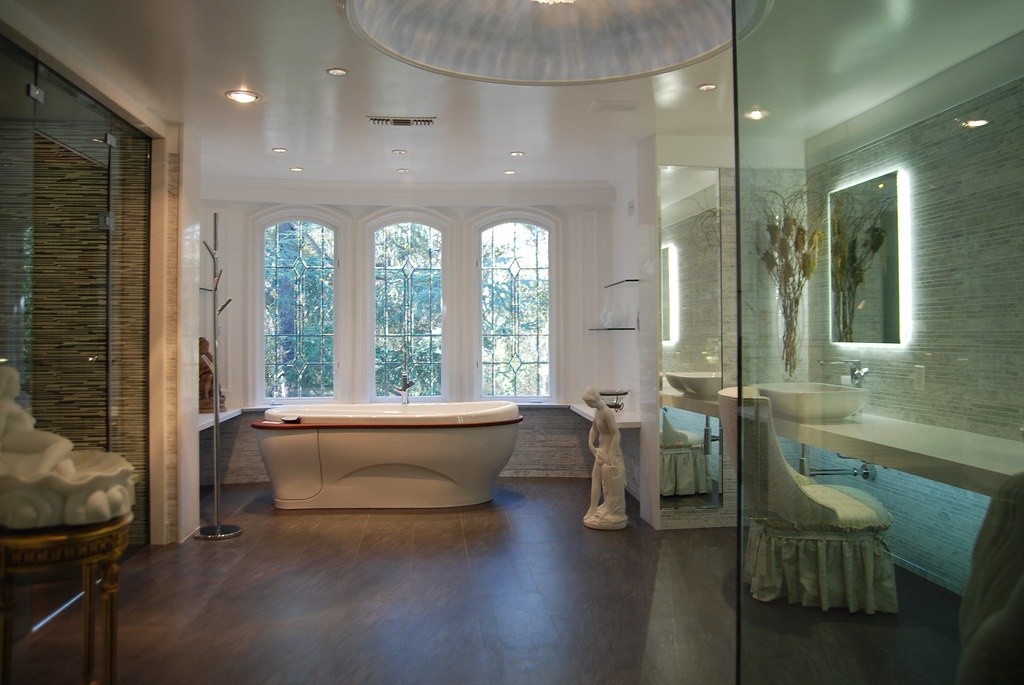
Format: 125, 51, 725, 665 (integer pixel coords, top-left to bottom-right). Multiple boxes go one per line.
744, 379, 868, 424
664, 371, 723, 401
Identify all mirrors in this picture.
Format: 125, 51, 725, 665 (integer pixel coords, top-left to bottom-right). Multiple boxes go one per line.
659, 165, 724, 512
830, 171, 900, 343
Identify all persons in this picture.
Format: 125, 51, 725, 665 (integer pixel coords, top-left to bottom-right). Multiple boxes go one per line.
199, 338, 224, 401
584, 385, 625, 521
1, 365, 97, 482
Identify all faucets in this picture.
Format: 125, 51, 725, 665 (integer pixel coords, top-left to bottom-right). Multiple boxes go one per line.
392, 370, 416, 404
818, 359, 869, 388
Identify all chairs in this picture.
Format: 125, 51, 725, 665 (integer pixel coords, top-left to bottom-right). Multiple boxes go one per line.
716, 385, 898, 612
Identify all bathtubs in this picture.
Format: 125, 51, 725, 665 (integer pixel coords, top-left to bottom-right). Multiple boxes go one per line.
249, 400, 524, 511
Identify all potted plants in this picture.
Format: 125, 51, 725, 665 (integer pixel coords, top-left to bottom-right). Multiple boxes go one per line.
830, 192, 897, 342
742, 172, 828, 382
